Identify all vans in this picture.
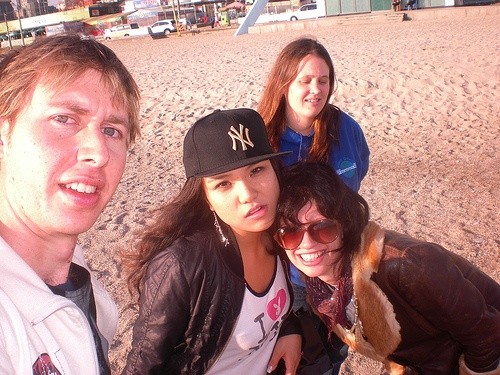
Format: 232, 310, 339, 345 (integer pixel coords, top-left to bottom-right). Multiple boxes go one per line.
103, 23, 139, 40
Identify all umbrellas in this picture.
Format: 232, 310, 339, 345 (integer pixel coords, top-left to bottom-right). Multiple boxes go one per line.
225, 1, 244, 15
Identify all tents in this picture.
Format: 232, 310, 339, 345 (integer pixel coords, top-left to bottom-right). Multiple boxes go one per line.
0, 27, 45, 41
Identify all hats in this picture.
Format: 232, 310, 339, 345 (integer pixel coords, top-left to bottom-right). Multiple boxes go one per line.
182, 108, 294, 178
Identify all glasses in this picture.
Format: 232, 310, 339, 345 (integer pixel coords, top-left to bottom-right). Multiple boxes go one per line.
273, 219, 342, 250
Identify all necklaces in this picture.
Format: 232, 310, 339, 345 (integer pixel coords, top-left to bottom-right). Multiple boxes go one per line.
343, 292, 359, 333
287, 119, 315, 132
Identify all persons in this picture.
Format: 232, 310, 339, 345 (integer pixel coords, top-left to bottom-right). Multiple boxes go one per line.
256, 38, 370, 316
189, 13, 219, 29
121, 108, 304, 375
0, 31, 142, 375
266, 161, 500, 375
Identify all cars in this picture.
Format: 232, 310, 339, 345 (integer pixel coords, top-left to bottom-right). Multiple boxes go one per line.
147, 19, 178, 35
286, 3, 318, 20
